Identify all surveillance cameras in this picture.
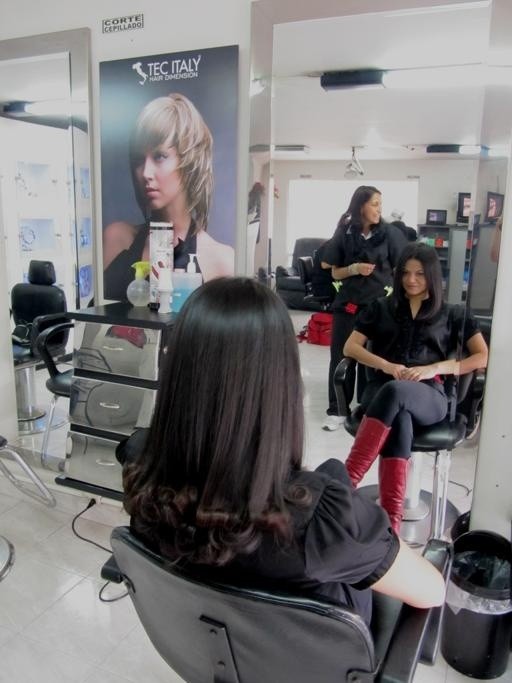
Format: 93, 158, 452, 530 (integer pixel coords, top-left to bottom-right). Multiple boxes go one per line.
350, 158, 366, 177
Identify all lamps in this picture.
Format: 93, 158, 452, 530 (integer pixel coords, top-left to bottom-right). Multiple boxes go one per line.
343, 145, 366, 181
245, 0, 512, 556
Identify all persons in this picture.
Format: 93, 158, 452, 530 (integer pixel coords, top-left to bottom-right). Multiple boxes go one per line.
309, 185, 419, 433
101, 91, 235, 307
121, 276, 447, 631
342, 242, 489, 535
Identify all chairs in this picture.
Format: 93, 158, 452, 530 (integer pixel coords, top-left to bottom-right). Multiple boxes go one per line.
274, 236, 334, 312
96, 524, 450, 682
326, 314, 491, 551
7, 256, 100, 470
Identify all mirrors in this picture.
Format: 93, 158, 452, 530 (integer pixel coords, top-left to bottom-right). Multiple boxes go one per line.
1, 26, 101, 476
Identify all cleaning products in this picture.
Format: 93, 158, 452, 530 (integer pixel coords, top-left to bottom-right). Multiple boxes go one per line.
126, 261, 149, 307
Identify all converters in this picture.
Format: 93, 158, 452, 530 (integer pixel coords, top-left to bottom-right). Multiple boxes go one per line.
100, 555, 123, 585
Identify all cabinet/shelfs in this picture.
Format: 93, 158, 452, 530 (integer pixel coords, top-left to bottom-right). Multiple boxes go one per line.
53, 302, 180, 508
417, 223, 452, 303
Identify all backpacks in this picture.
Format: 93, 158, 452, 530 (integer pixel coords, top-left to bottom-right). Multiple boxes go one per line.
298, 312, 335, 346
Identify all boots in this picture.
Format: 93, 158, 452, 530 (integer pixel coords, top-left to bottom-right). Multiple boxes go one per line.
377, 455, 412, 539
344, 412, 392, 491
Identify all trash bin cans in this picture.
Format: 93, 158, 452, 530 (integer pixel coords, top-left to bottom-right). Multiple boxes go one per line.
439, 530, 512, 679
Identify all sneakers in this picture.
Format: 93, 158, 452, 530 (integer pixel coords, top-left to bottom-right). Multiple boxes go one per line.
321, 414, 346, 432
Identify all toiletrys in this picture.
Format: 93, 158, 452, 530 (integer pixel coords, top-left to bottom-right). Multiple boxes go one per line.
185, 252, 202, 287
